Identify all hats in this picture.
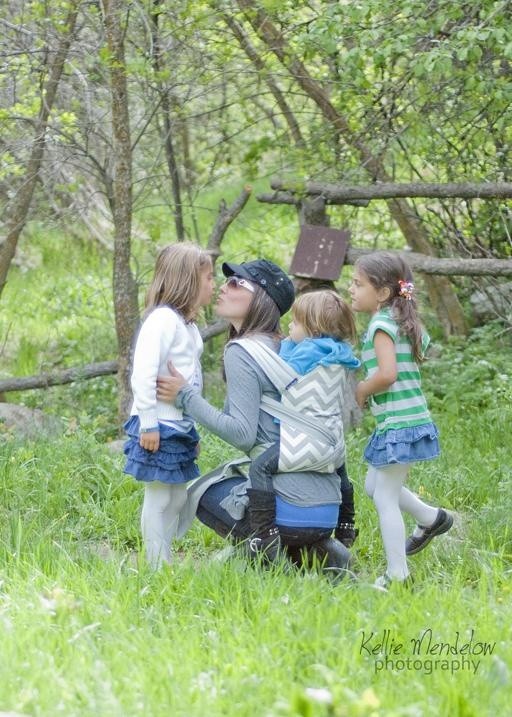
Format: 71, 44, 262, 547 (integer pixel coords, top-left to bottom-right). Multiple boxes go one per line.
222, 257, 296, 317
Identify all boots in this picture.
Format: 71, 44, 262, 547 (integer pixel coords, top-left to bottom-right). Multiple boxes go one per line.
242, 488, 281, 563
334, 484, 357, 549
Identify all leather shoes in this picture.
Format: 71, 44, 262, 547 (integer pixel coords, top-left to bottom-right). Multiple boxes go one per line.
373, 570, 415, 593
406, 507, 453, 555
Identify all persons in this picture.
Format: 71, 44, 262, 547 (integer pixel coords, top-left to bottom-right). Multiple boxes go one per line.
122, 237, 453, 590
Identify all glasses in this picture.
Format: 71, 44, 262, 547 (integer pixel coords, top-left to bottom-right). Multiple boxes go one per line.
223, 276, 256, 295
